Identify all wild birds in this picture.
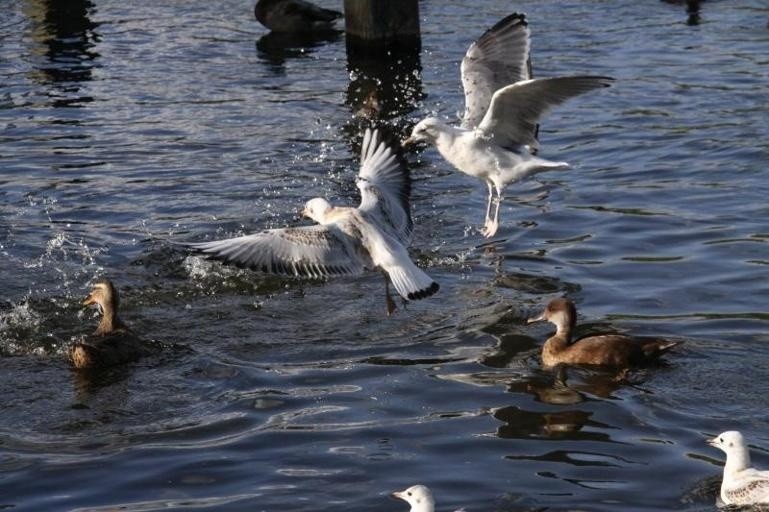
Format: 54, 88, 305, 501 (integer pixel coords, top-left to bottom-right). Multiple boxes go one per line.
166, 126, 441, 317
700, 430, 769, 508
402, 12, 616, 238
253, 0, 344, 34
386, 483, 462, 512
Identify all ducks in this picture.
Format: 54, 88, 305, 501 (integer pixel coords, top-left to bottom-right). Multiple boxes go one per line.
528, 297, 688, 367
66, 277, 139, 371
